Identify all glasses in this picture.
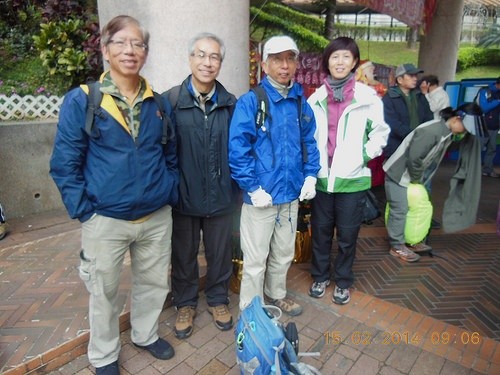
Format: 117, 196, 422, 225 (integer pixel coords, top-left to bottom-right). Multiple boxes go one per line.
109, 40, 148, 51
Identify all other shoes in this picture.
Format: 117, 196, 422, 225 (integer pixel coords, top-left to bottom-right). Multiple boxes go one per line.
133, 338, 175, 360
96, 361, 121, 375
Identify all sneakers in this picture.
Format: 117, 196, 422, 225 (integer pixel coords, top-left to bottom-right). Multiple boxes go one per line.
206, 303, 232, 329
331, 285, 350, 304
174, 307, 197, 336
389, 245, 420, 262
307, 278, 330, 298
266, 294, 301, 316
408, 243, 431, 251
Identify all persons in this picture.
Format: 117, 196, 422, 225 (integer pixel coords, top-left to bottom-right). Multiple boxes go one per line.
382, 101, 491, 260
50, 15, 179, 375
307, 37, 392, 304
0, 201, 9, 240
473, 77, 500, 178
381, 63, 449, 228
160, 32, 239, 339
228, 35, 321, 326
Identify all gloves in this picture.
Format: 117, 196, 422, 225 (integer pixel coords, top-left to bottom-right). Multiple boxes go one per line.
300, 175, 318, 201
248, 185, 273, 209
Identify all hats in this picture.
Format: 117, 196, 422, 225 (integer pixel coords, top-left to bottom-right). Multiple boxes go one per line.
458, 110, 488, 138
263, 36, 299, 63
394, 63, 424, 78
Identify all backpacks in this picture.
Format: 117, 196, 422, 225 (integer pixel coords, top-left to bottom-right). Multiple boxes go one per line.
235, 295, 320, 375
